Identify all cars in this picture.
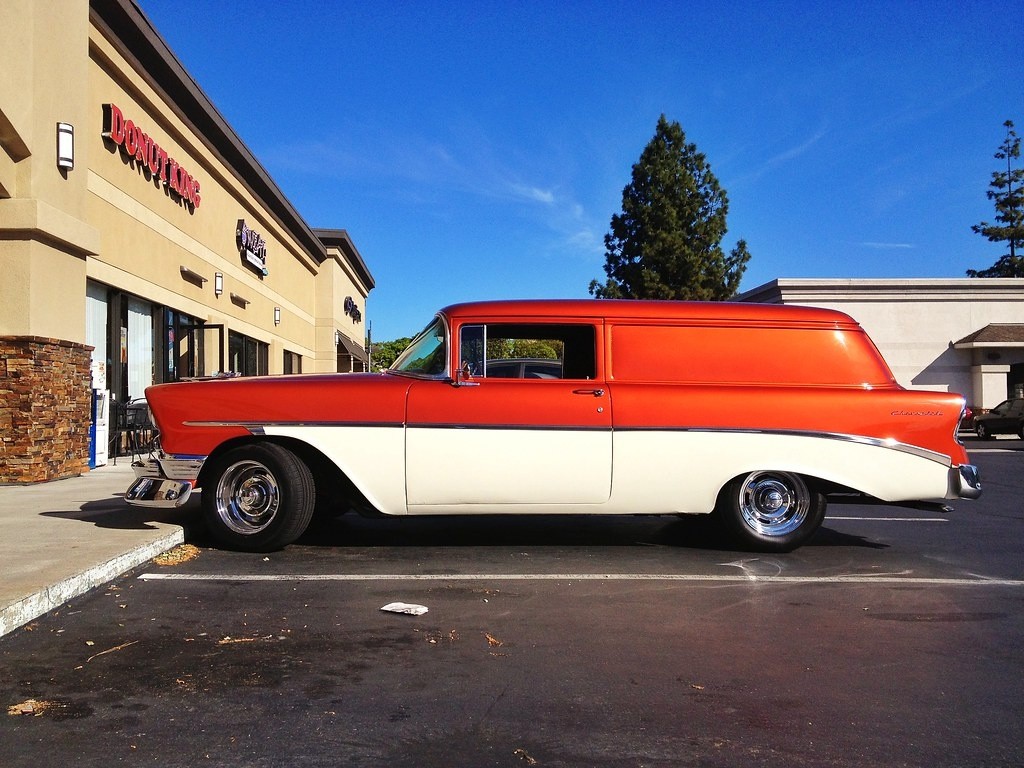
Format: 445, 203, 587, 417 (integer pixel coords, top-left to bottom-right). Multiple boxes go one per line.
439, 358, 566, 381
973, 397, 1024, 440
124, 299, 985, 554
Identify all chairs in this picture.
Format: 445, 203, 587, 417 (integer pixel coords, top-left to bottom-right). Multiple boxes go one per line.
108, 397, 160, 467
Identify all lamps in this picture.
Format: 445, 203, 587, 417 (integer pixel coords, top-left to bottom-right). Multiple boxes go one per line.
215, 272, 223, 295
335, 332, 339, 344
56, 121, 74, 172
275, 307, 280, 324
